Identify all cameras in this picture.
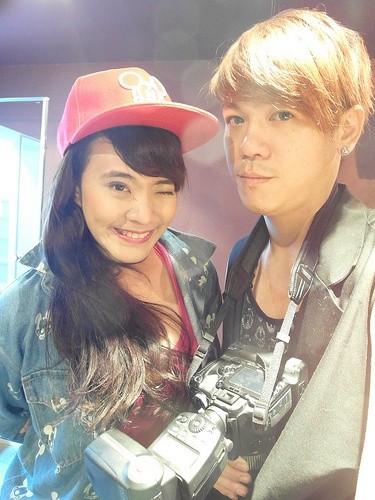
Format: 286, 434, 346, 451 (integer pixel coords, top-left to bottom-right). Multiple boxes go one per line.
84, 342, 310, 500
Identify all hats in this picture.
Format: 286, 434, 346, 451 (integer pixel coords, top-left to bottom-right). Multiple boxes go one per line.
57, 66, 219, 155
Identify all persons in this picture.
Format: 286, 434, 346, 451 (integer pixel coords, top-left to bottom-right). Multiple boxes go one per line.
209, 7, 375, 500
0, 67, 224, 500
